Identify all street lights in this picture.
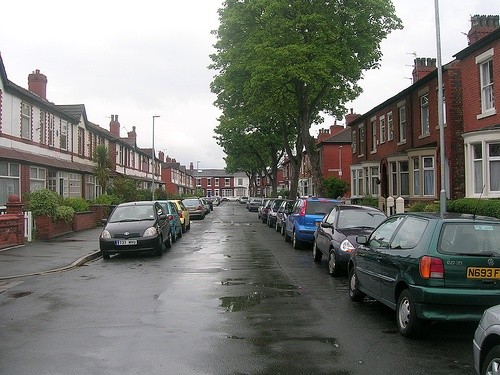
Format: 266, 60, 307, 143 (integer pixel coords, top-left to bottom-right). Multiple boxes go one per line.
197, 161, 201, 196
152, 116, 160, 200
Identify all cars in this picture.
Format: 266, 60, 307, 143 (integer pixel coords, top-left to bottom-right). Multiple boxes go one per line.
99, 201, 172, 260
167, 200, 190, 234
312, 204, 389, 278
347, 211, 500, 339
155, 200, 183, 244
265, 198, 284, 228
226, 196, 240, 202
273, 200, 294, 236
285, 196, 343, 249
239, 196, 278, 223
473, 304, 500, 375
182, 196, 223, 220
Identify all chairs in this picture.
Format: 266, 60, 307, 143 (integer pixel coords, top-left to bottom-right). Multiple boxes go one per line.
451, 234, 478, 251
